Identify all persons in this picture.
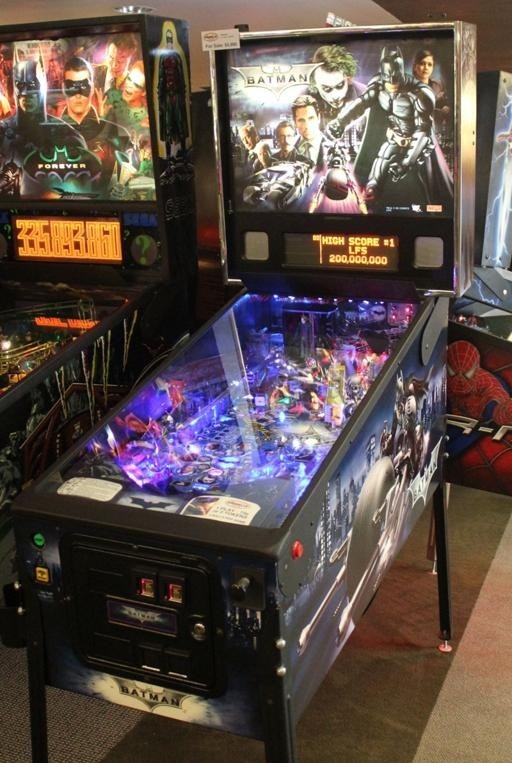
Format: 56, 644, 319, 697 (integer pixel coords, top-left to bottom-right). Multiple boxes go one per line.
231, 45, 454, 214
0, 20, 192, 201
443, 340, 512, 496
